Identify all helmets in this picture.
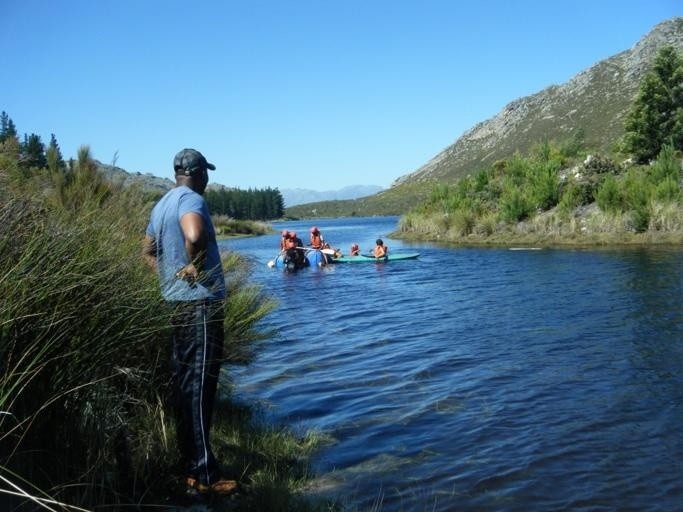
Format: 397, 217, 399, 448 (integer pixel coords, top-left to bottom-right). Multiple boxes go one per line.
376, 239, 383, 245
282, 231, 296, 239
311, 227, 317, 233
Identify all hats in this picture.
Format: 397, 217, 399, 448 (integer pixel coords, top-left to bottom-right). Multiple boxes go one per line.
174, 148, 216, 176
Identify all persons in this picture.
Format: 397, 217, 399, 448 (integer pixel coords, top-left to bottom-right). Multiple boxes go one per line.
374, 239, 387, 257
310, 227, 324, 250
142, 148, 239, 495
281, 231, 290, 250
286, 232, 298, 250
350, 244, 360, 256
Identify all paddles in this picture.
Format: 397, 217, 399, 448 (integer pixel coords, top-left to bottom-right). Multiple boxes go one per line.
296, 247, 335, 255
268, 250, 282, 268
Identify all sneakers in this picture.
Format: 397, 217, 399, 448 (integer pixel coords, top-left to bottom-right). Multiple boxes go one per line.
186, 479, 238, 496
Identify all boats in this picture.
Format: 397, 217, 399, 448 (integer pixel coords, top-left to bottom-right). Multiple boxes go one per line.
275, 246, 421, 274
